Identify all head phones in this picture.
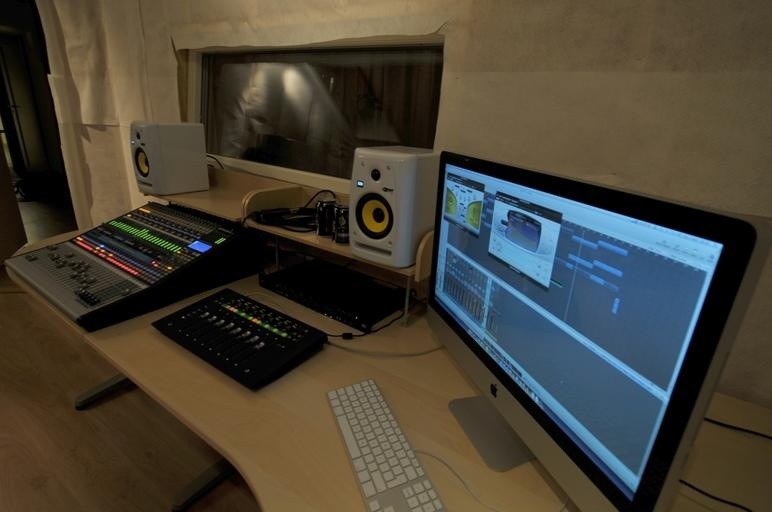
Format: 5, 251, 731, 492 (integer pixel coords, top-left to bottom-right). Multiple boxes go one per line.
251, 207, 316, 232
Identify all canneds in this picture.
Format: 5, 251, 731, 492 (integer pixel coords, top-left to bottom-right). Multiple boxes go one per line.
315, 199, 349, 244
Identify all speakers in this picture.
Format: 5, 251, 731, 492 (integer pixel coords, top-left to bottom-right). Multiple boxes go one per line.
129, 121, 209, 195
348, 146, 440, 268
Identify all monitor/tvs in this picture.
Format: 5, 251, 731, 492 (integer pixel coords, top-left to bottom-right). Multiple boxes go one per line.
427, 149, 772, 512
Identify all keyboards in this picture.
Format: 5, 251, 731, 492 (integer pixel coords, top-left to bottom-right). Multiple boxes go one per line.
325, 378, 447, 512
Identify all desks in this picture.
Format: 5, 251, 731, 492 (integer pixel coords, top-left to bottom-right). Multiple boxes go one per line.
8, 168, 771, 512
